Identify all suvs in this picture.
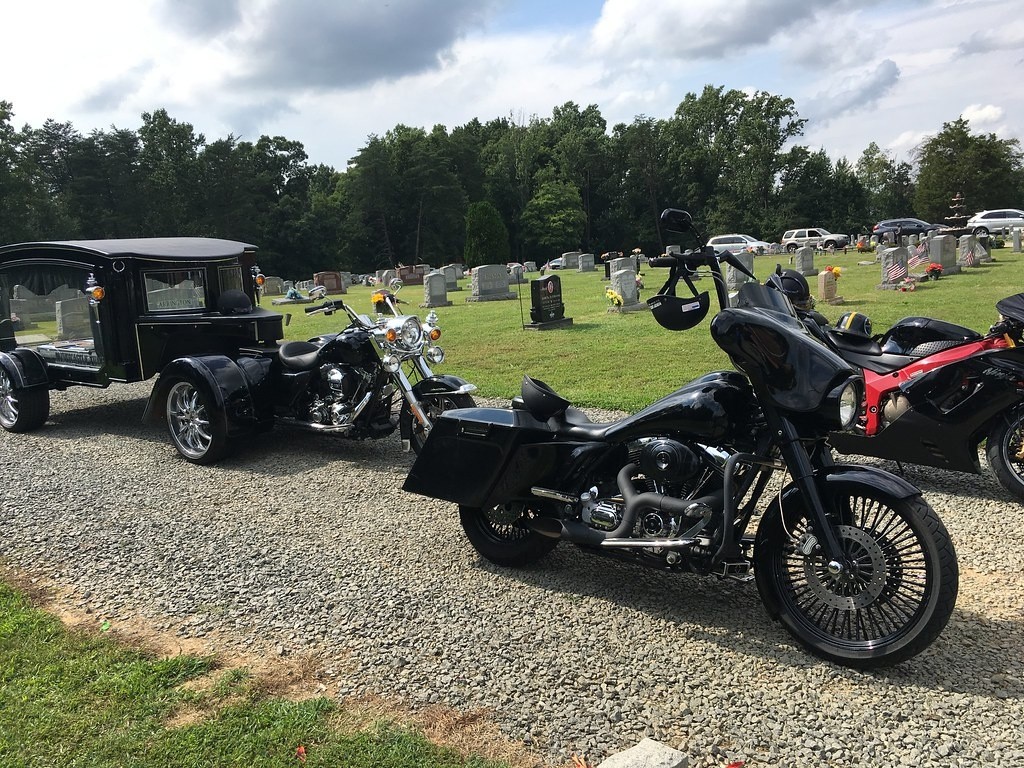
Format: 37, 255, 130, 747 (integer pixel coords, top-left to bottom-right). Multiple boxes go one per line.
706, 234, 770, 256
872, 218, 942, 246
781, 228, 850, 253
966, 208, 1024, 237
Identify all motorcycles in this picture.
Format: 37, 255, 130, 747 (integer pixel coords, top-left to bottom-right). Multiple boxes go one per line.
398, 209, 958, 672
728, 264, 1024, 502
138, 276, 480, 470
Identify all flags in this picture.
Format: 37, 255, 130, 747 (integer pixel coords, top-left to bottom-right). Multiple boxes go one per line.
908, 250, 931, 270
915, 242, 929, 254
887, 259, 906, 280
966, 247, 974, 265
548, 260, 551, 269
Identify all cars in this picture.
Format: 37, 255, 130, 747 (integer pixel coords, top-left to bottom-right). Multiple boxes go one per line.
539, 257, 563, 276
